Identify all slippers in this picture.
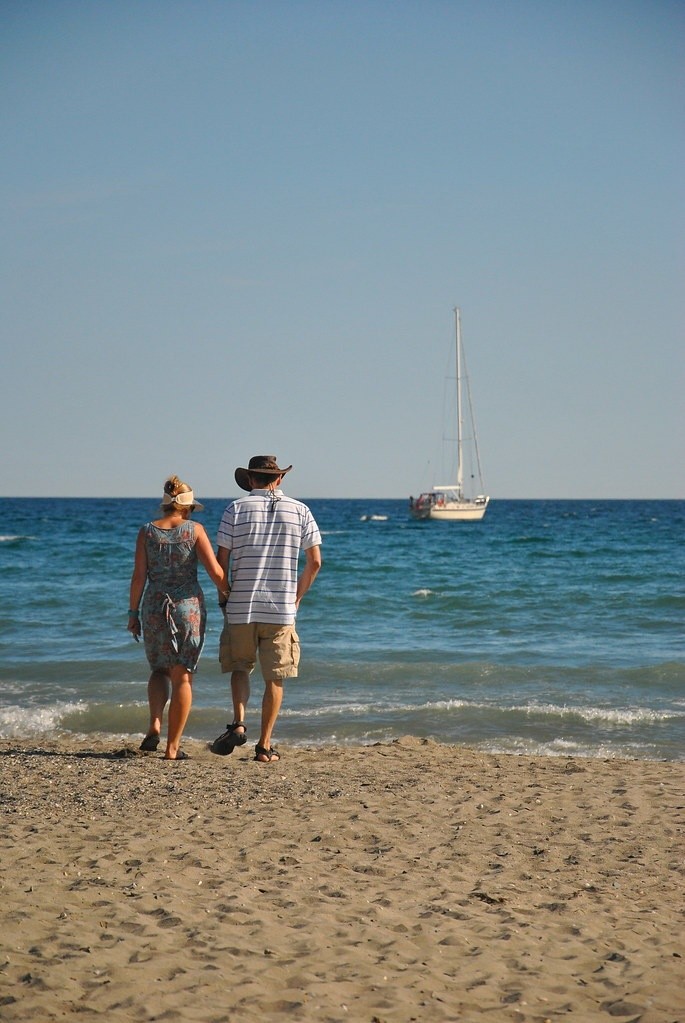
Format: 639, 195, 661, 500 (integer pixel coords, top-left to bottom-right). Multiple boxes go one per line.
165, 750, 188, 760
139, 735, 159, 751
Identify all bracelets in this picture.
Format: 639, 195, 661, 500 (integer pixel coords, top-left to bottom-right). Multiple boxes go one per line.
128, 609, 139, 617
222, 589, 231, 597
218, 601, 228, 607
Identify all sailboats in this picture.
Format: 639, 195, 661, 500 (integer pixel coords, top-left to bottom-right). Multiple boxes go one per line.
410, 307, 489, 520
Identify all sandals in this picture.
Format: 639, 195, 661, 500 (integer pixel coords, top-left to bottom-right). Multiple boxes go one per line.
253, 743, 280, 762
213, 722, 247, 755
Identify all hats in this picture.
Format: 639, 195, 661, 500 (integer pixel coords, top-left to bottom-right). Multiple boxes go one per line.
235, 456, 292, 492
160, 489, 205, 512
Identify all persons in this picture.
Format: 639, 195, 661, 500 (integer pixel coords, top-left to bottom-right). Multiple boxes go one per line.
127, 477, 231, 760
209, 455, 323, 761
